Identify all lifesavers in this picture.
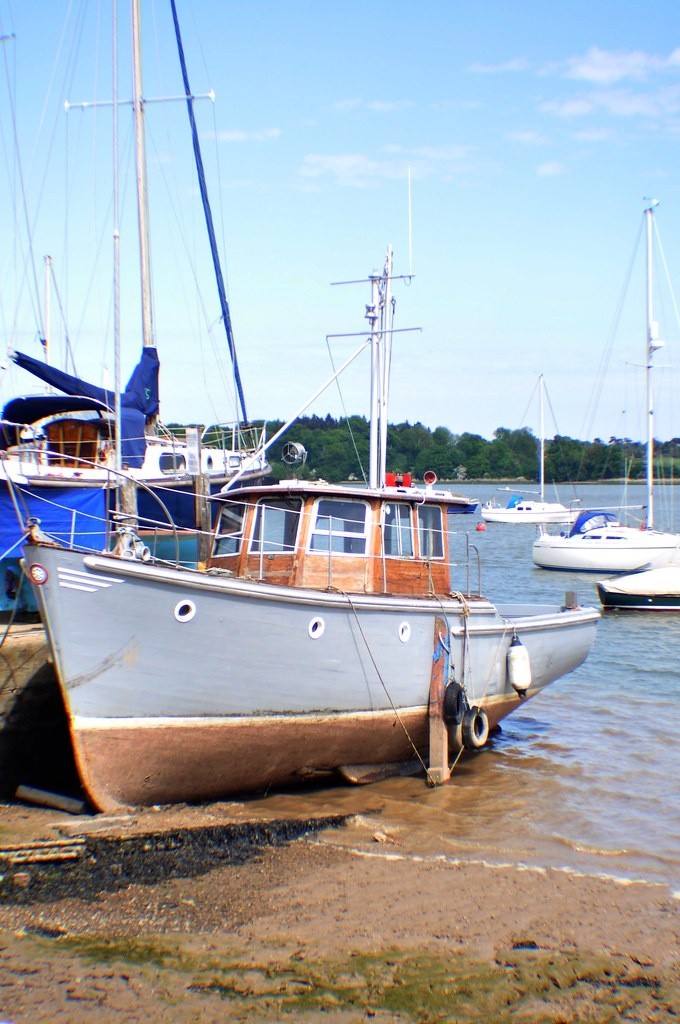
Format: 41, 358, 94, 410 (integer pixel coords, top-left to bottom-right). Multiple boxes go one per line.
445, 681, 489, 752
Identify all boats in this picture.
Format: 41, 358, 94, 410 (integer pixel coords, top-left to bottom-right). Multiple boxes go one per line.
0, 170, 602, 811
594, 560, 680, 613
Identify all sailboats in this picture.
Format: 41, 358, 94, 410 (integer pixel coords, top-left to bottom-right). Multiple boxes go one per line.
479, 373, 588, 523
529, 197, 680, 574
1, 0, 276, 616
